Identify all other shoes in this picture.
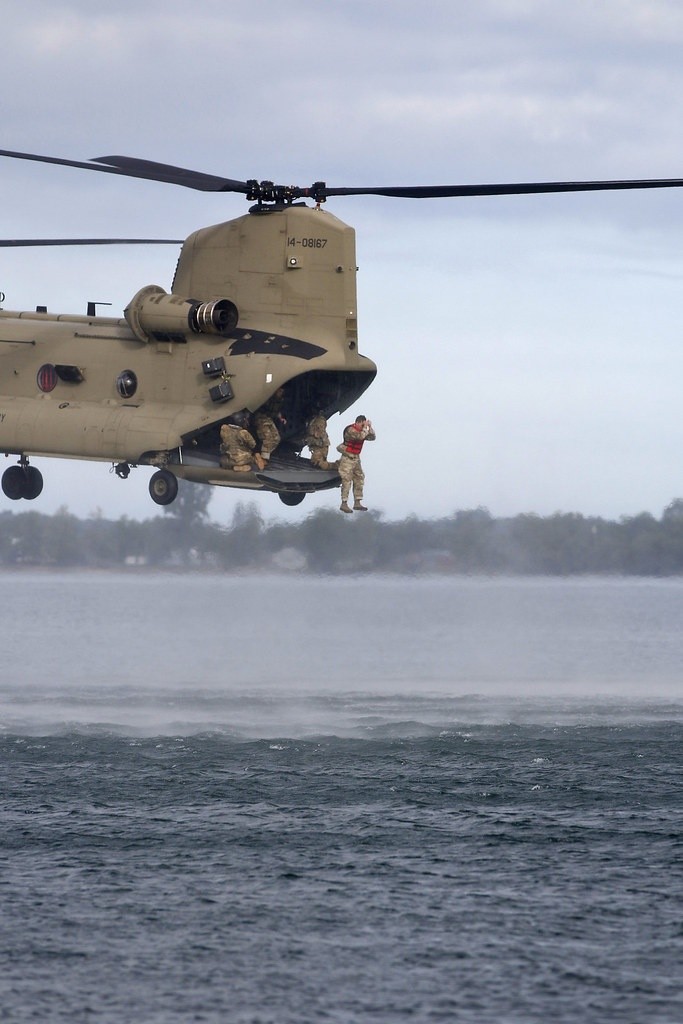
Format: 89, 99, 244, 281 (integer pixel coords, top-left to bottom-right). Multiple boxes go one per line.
234, 465, 251, 472
254, 453, 264, 470
262, 458, 268, 466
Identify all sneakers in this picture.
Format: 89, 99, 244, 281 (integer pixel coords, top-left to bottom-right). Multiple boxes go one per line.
353, 501, 367, 511
340, 501, 353, 513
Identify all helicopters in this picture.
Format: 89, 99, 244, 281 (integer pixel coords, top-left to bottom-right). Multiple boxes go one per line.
0, 150, 683, 505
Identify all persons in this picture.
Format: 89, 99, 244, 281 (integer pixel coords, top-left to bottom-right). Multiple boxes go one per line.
219, 412, 264, 472
253, 385, 287, 471
336, 415, 377, 513
299, 406, 342, 470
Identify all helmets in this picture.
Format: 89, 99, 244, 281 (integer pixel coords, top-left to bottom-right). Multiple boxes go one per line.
231, 412, 246, 427
